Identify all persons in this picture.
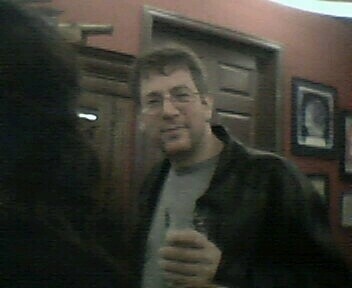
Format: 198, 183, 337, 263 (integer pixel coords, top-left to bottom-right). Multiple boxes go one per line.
127, 44, 340, 287
0, 0, 139, 288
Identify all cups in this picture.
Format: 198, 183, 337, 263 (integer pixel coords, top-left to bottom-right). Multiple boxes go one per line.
164, 207, 209, 288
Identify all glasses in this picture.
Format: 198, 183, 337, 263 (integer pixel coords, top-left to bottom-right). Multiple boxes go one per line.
139, 87, 201, 115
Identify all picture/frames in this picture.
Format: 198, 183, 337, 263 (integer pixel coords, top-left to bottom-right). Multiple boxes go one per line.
338, 108, 352, 185
338, 191, 352, 229
290, 75, 341, 161
305, 170, 331, 220
